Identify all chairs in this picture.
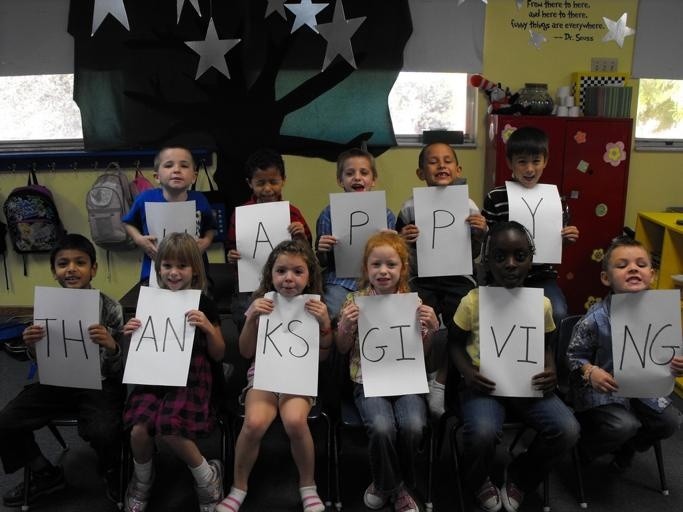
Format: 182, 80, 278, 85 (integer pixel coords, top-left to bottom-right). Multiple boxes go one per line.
23, 314, 128, 512
333, 299, 438, 512
441, 303, 552, 512
128, 309, 239, 512
552, 315, 673, 510
229, 311, 333, 511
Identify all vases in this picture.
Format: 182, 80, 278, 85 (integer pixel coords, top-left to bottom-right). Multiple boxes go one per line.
515, 83, 553, 117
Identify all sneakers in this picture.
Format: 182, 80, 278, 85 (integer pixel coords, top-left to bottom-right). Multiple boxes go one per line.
501, 480, 525, 512
393, 486, 420, 512
2, 463, 68, 508
476, 475, 502, 512
195, 458, 226, 512
123, 468, 157, 512
363, 481, 387, 509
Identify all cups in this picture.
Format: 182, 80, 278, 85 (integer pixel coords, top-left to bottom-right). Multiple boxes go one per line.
569, 106, 580, 116
564, 96, 575, 107
556, 106, 568, 116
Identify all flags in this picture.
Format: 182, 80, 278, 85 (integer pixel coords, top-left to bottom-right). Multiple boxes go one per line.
68, 0, 413, 162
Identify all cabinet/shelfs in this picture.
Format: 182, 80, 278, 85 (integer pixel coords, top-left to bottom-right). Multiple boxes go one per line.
632, 210, 683, 402
483, 115, 634, 315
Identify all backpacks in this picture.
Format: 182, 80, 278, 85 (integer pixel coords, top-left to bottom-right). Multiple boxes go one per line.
0, 315, 34, 360
4, 166, 66, 275
85, 162, 139, 286
0, 221, 10, 292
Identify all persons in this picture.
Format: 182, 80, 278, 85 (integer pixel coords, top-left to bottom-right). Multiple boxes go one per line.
216, 240, 333, 512
333, 231, 439, 512
315, 147, 396, 319
123, 233, 225, 512
225, 152, 313, 324
120, 144, 219, 286
449, 222, 581, 512
396, 140, 489, 417
565, 241, 682, 498
0, 234, 124, 506
481, 127, 579, 339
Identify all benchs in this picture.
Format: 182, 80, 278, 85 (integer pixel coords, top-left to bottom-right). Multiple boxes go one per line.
119, 259, 588, 322
